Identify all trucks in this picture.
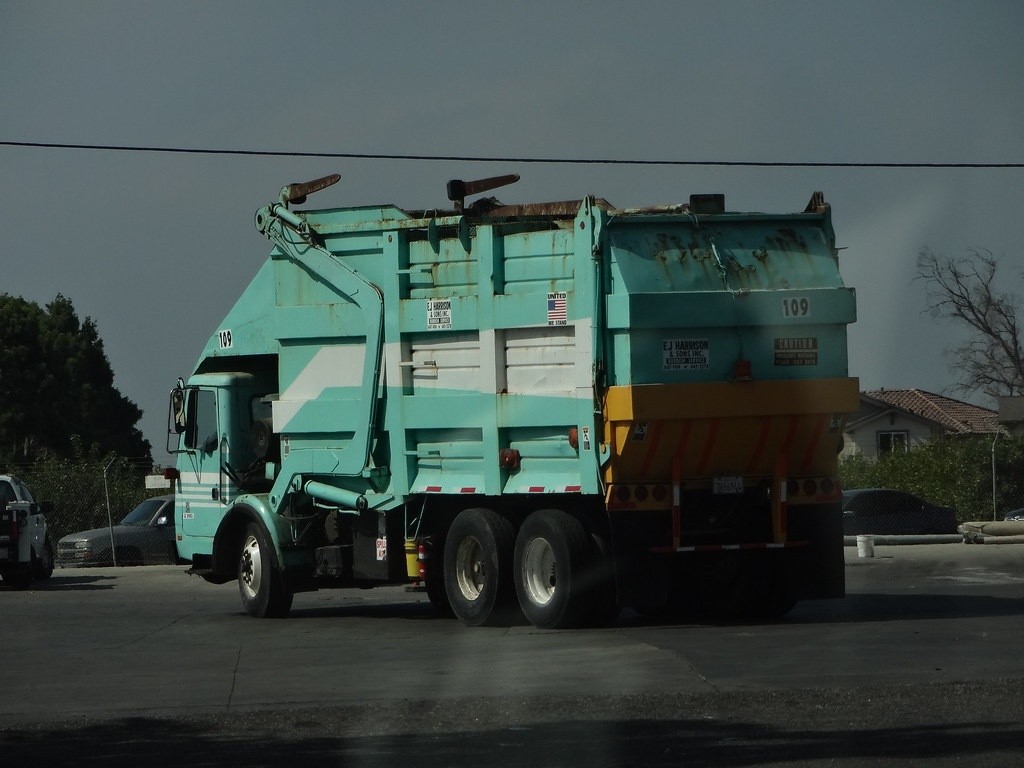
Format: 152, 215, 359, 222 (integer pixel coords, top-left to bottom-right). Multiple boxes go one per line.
164, 169, 859, 630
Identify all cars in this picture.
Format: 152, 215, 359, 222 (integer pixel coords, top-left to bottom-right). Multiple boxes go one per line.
841, 488, 960, 538
0, 473, 56, 590
57, 492, 176, 569
